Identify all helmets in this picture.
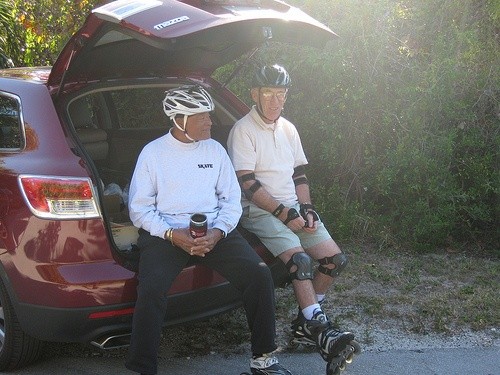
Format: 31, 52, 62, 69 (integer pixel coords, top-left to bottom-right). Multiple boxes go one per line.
162, 85, 215, 117
251, 64, 292, 88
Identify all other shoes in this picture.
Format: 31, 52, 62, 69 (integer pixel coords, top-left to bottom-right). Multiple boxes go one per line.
240, 355, 293, 375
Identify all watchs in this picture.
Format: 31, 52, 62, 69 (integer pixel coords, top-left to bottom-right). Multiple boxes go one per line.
215, 228, 226, 239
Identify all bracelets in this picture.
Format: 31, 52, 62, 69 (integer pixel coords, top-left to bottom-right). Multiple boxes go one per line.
166, 228, 175, 247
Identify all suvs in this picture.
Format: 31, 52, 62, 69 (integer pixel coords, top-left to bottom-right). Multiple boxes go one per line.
0, 0, 342, 369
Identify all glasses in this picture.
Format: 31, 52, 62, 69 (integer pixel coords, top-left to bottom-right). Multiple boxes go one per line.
261, 91, 287, 101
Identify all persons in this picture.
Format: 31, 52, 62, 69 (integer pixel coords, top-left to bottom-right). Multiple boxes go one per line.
227, 63, 361, 375
125, 85, 294, 375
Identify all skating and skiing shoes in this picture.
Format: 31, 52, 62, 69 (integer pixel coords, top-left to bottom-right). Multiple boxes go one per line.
305, 318, 362, 375
288, 307, 321, 354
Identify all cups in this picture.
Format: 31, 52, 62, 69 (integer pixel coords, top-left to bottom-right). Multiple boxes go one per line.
190, 213, 207, 239
